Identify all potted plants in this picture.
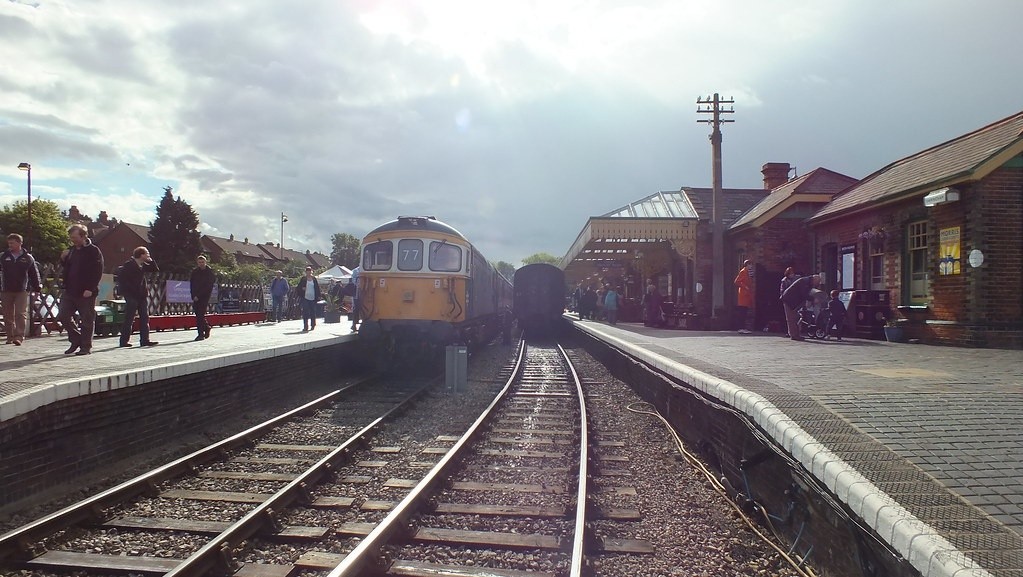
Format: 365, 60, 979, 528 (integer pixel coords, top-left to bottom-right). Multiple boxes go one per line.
884, 320, 904, 342
322, 288, 342, 323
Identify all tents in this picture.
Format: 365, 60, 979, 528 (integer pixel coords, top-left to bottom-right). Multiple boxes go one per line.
316, 265, 353, 285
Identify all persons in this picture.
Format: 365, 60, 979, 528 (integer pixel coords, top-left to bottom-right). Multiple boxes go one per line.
576, 283, 625, 325
734, 260, 753, 334
824, 290, 846, 342
118, 246, 160, 347
332, 278, 356, 301
270, 271, 288, 323
351, 277, 360, 331
781, 275, 821, 340
645, 285, 659, 327
297, 267, 320, 331
502, 305, 512, 346
60, 224, 103, 355
781, 267, 796, 337
190, 256, 215, 341
0, 233, 41, 345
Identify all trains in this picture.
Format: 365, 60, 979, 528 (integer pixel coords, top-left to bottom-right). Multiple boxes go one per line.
511, 262, 568, 342
352, 214, 514, 371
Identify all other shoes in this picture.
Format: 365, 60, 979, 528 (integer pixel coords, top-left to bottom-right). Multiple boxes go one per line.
16, 340, 21, 345
303, 329, 308, 331
311, 324, 316, 329
838, 338, 842, 341
121, 344, 132, 347
738, 329, 752, 334
824, 336, 830, 340
205, 326, 211, 338
65, 343, 80, 353
195, 335, 205, 340
76, 351, 88, 355
144, 342, 158, 346
351, 326, 356, 332
798, 337, 804, 340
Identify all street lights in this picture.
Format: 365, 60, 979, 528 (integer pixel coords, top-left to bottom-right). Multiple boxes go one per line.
280, 211, 289, 262
18, 162, 36, 337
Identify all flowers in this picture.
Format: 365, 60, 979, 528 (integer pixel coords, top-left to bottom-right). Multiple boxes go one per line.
859, 225, 885, 242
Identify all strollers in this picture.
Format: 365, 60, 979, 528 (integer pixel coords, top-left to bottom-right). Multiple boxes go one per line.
796, 296, 831, 340
